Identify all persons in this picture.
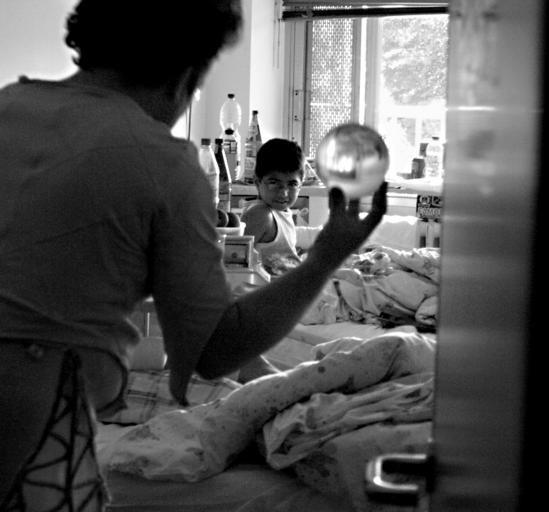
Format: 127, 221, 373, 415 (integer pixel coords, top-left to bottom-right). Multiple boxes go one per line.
238, 137, 309, 282
1, 1, 390, 511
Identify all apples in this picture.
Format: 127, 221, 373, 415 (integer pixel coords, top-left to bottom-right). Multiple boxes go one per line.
227, 211, 241, 227
216, 208, 228, 226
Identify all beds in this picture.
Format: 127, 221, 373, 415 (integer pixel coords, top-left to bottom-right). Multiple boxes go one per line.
94, 245, 441, 511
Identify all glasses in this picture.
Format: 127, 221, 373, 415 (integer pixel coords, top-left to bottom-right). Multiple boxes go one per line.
261, 178, 302, 192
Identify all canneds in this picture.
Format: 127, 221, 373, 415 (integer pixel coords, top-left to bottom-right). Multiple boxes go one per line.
411, 158, 425, 178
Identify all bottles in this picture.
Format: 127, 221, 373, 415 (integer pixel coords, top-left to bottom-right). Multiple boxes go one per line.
197, 93, 242, 212
424, 136, 444, 186
245, 110, 262, 182
